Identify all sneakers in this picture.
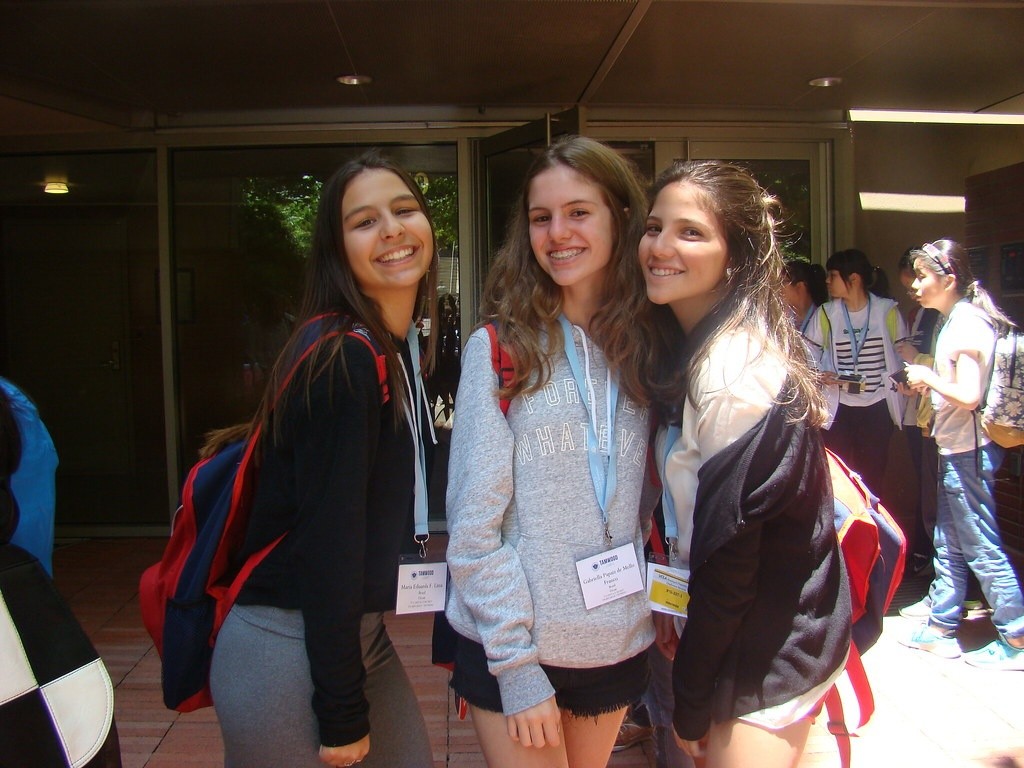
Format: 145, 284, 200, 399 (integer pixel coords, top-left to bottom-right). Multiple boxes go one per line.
612, 718, 652, 751
899, 621, 965, 660
963, 639, 1024, 672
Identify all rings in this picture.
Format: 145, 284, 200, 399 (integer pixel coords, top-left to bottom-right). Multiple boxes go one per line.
344, 760, 361, 767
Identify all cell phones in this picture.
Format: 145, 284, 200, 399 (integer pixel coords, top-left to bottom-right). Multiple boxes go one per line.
888, 367, 908, 386
834, 375, 865, 384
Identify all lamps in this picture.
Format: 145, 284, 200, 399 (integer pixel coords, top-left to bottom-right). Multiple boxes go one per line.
34, 167, 70, 196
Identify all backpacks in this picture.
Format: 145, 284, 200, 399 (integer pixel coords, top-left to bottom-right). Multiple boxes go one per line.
818, 444, 908, 768
948, 309, 1024, 448
139, 312, 388, 714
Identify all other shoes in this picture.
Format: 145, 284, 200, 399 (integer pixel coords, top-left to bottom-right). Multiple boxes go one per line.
962, 597, 984, 612
906, 559, 932, 578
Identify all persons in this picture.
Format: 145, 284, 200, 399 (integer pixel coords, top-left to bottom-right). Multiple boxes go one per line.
432, 137, 685, 768
209, 155, 439, 768
0, 371, 123, 768
638, 160, 852, 768
780, 239, 1024, 672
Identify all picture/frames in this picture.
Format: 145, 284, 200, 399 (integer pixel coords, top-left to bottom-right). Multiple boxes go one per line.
152, 269, 197, 325
